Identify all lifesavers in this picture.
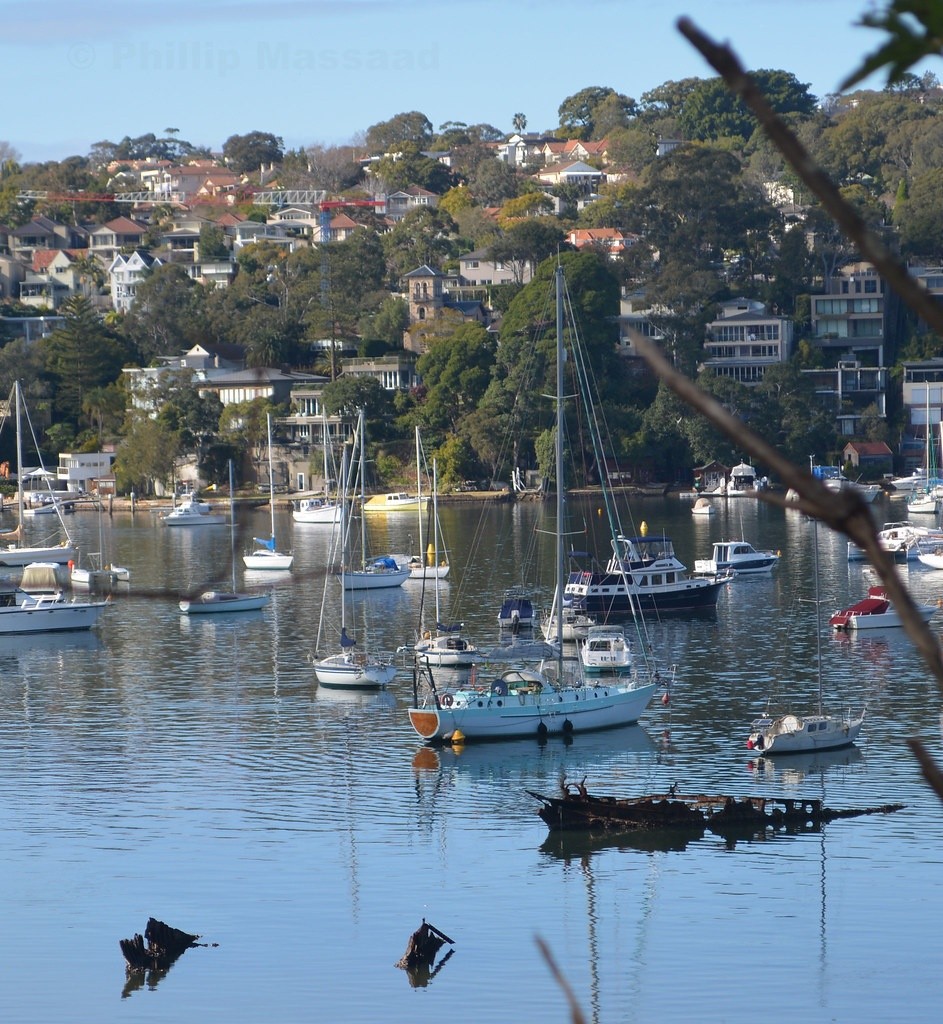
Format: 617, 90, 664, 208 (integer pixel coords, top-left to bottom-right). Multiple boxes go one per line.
934, 547, 942, 556
440, 693, 454, 706
726, 569, 736, 578
518, 691, 526, 706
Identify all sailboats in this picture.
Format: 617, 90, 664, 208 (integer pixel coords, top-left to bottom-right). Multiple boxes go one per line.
319, 405, 416, 592
411, 719, 663, 1023
241, 569, 296, 701
0, 381, 79, 567
313, 683, 396, 883
396, 238, 665, 740
243, 413, 296, 569
747, 741, 869, 968
737, 512, 872, 757
386, 422, 455, 579
412, 459, 478, 666
181, 609, 268, 722
292, 404, 346, 523
305, 407, 399, 688
178, 458, 273, 613
72, 448, 118, 588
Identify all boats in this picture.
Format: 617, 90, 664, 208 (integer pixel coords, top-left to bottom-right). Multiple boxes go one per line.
524, 778, 825, 833
692, 498, 716, 515
804, 377, 943, 572
158, 488, 228, 526
0, 587, 118, 634
497, 586, 537, 630
565, 533, 739, 615
360, 491, 430, 512
829, 582, 937, 628
538, 605, 595, 640
580, 625, 632, 671
693, 540, 782, 576
531, 827, 784, 863
727, 462, 763, 497
